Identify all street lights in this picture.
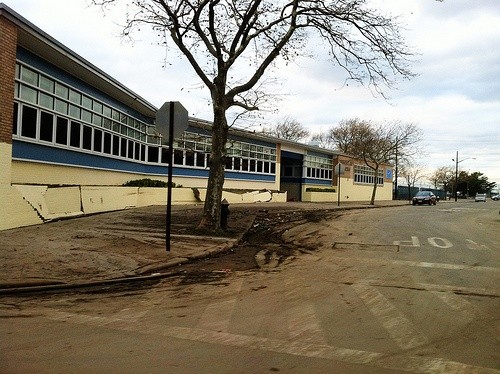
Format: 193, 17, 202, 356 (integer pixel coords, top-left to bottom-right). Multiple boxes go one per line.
452, 157, 476, 202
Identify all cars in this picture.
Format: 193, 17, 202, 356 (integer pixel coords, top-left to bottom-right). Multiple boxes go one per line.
412, 191, 437, 206
475, 194, 486, 202
490, 194, 500, 201
